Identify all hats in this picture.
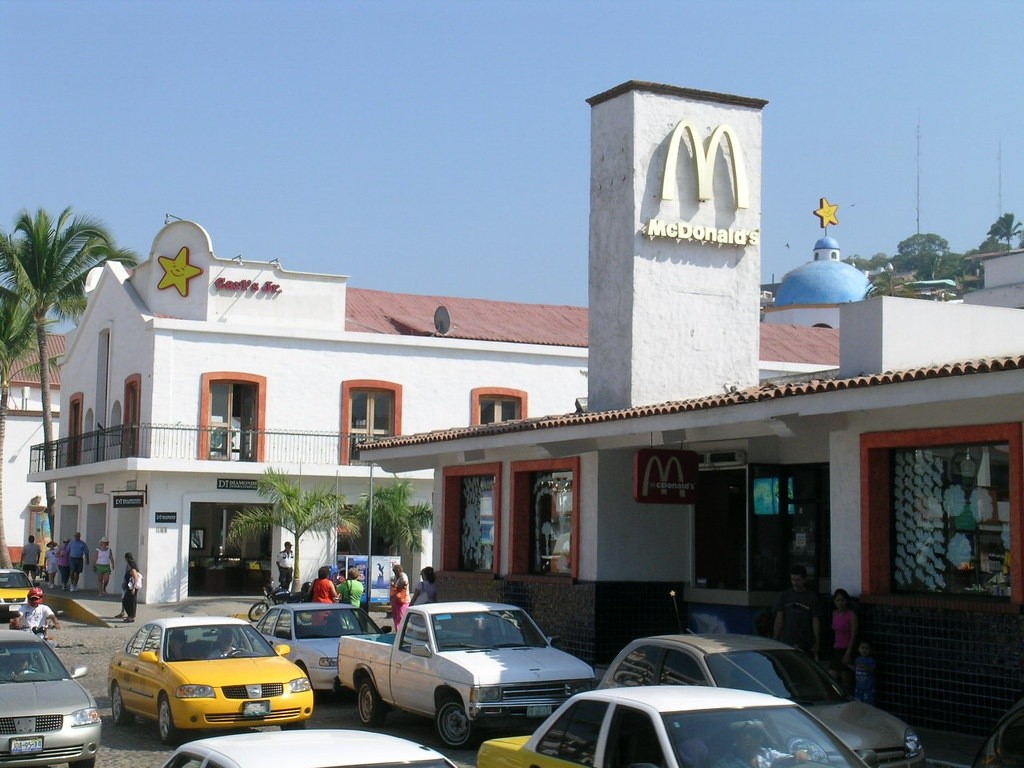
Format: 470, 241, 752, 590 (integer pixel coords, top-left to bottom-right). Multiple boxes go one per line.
46, 541, 58, 548
100, 537, 108, 543
285, 542, 293, 548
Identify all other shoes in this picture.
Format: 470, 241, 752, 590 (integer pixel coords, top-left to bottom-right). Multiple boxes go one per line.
75, 586, 79, 591
48, 582, 54, 588
115, 613, 125, 618
62, 584, 67, 590
122, 619, 135, 623
70, 585, 75, 591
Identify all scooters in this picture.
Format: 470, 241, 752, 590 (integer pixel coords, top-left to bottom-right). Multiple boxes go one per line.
8, 610, 65, 649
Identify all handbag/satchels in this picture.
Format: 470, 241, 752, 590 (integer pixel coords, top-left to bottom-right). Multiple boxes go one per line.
338, 598, 351, 605
413, 582, 429, 606
304, 579, 318, 603
390, 575, 406, 605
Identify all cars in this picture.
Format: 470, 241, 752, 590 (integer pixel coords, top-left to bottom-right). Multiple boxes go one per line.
242, 601, 393, 705
594, 632, 928, 768
108, 616, 315, 745
476, 683, 869, 768
159, 729, 458, 768
0, 567, 39, 622
0, 627, 103, 768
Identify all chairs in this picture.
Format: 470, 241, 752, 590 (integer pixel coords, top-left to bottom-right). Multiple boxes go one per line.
472, 617, 495, 649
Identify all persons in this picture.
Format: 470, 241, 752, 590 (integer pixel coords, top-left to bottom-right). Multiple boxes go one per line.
44, 532, 90, 592
299, 565, 364, 624
15, 587, 61, 639
9, 653, 40, 680
208, 630, 244, 659
93, 536, 115, 596
164, 629, 195, 662
19, 535, 42, 587
276, 542, 294, 592
5, 576, 25, 587
390, 565, 411, 632
772, 564, 887, 703
409, 566, 438, 606
710, 732, 809, 768
114, 552, 143, 623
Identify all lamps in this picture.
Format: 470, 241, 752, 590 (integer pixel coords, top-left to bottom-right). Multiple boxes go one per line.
164, 213, 182, 225
269, 258, 279, 269
231, 255, 243, 266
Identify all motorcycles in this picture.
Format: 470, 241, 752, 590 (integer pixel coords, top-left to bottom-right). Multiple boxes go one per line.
247, 578, 312, 622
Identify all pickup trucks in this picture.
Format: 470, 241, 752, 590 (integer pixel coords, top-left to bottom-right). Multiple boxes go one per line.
332, 600, 602, 751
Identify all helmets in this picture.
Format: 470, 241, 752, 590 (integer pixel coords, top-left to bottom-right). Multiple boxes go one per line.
28, 587, 45, 606
